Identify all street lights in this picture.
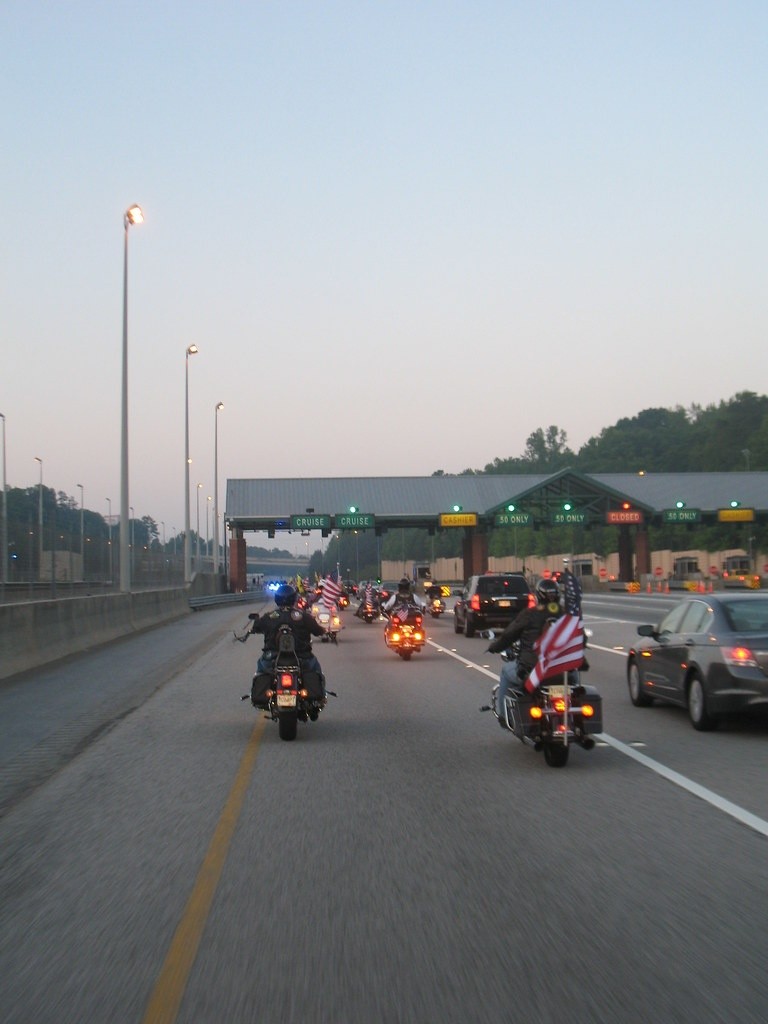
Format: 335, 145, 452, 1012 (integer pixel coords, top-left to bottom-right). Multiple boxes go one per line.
119, 203, 146, 598
0, 343, 225, 584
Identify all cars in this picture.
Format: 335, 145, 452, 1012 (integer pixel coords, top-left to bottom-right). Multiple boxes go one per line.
725, 569, 759, 582
453, 574, 538, 638
343, 580, 400, 603
626, 593, 768, 732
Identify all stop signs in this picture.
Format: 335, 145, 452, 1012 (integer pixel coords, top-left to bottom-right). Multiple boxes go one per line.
543, 566, 718, 579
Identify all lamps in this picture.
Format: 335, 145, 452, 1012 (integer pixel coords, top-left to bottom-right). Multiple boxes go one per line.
453, 505, 463, 513
676, 501, 687, 510
730, 501, 741, 509
349, 506, 360, 513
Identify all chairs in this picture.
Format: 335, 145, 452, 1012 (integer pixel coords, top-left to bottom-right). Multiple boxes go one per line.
733, 618, 750, 630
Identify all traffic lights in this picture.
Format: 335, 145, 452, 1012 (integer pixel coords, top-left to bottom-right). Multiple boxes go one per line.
454, 506, 460, 511
623, 503, 630, 510
676, 501, 684, 509
564, 504, 571, 511
731, 501, 738, 507
350, 506, 356, 513
508, 504, 515, 512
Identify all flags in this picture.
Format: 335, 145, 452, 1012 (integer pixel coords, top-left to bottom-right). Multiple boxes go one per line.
303, 577, 310, 591
397, 603, 409, 622
322, 574, 342, 609
336, 576, 344, 591
366, 580, 372, 606
525, 567, 586, 693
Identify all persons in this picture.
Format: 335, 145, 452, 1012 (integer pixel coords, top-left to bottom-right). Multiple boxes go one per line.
489, 580, 589, 727
307, 579, 327, 605
263, 580, 295, 587
353, 581, 381, 617
425, 579, 446, 612
384, 579, 424, 631
336, 585, 349, 600
252, 585, 324, 675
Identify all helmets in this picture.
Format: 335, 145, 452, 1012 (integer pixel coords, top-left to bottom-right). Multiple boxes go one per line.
275, 586, 297, 605
399, 578, 410, 587
536, 579, 560, 604
431, 579, 437, 584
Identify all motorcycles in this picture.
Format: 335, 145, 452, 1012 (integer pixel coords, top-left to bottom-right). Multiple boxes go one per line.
424, 589, 446, 619
380, 602, 427, 661
479, 628, 602, 769
240, 613, 338, 742
296, 590, 382, 643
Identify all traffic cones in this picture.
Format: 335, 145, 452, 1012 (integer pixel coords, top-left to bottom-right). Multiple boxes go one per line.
646, 581, 713, 594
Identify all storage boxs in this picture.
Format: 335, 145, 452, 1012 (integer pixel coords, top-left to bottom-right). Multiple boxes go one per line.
507, 691, 542, 738
302, 673, 326, 712
572, 685, 603, 735
250, 671, 274, 712
